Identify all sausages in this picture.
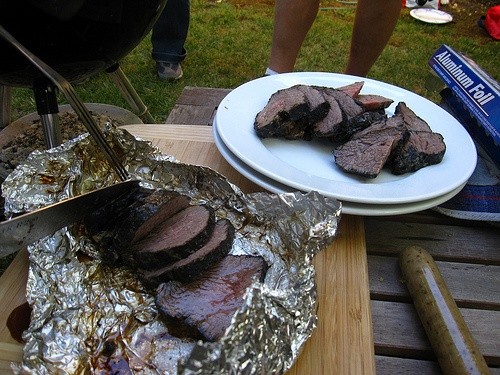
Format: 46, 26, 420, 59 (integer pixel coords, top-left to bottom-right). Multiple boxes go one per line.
398, 244, 491, 375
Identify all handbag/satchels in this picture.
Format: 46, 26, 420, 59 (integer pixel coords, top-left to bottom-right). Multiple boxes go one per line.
401, 0, 441, 10
478, 5, 500, 40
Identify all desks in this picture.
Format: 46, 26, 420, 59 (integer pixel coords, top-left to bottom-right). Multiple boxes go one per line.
163, 86, 500, 375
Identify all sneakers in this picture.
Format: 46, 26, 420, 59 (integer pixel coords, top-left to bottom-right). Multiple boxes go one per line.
156, 60, 183, 83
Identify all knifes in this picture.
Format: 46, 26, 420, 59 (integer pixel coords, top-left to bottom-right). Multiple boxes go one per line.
0, 180, 141, 257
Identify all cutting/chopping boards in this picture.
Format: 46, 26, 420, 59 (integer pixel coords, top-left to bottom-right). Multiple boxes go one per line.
0, 124, 376, 375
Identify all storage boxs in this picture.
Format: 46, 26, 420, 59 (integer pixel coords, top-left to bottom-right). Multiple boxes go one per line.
428, 44, 500, 144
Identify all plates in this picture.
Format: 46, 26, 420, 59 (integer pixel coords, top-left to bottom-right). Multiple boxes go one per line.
213, 72, 478, 216
410, 8, 453, 24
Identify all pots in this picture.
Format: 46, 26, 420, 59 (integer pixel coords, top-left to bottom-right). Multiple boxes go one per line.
0, 0, 167, 87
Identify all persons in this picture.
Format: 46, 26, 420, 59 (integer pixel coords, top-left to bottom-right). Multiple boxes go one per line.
150, 0, 190, 81
263, 0, 404, 79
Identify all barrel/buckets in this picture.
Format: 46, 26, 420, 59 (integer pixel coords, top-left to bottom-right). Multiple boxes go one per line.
483, 5, 500, 40
